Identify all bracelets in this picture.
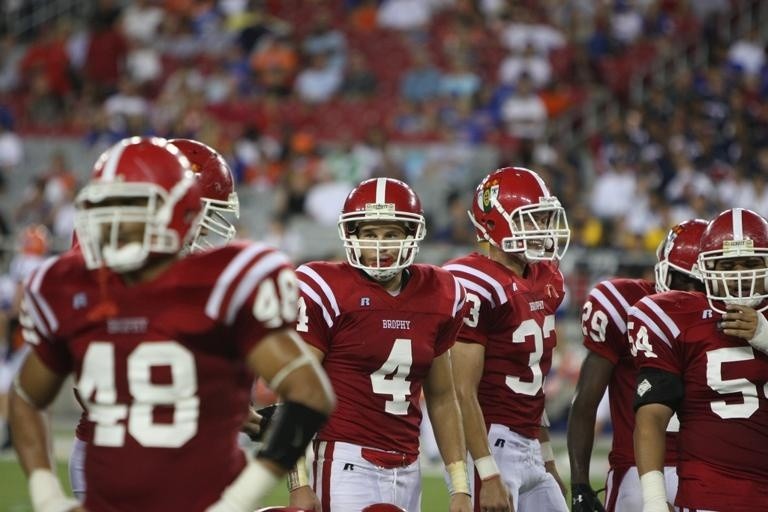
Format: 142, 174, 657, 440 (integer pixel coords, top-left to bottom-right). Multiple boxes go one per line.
541, 442, 555, 463
287, 456, 310, 493
474, 456, 499, 480
444, 461, 472, 497
255, 402, 326, 475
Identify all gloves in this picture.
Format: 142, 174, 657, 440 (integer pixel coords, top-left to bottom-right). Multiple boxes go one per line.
571, 483, 607, 512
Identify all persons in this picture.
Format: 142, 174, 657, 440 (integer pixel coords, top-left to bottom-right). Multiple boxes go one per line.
68, 139, 286, 502
1, 226, 49, 442
1, 2, 767, 245
1, 135, 336, 512
628, 207, 766, 510
297, 178, 473, 510
443, 168, 571, 512
568, 219, 711, 511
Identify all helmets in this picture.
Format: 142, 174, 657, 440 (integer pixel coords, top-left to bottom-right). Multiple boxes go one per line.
343, 177, 424, 261
90, 136, 234, 256
660, 207, 768, 295
471, 166, 555, 264
